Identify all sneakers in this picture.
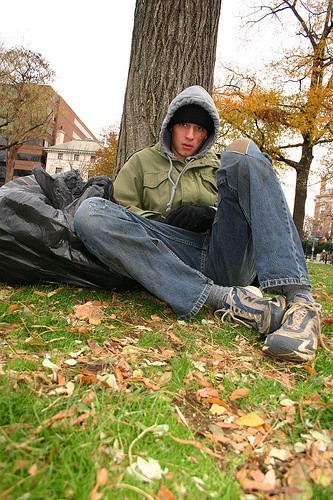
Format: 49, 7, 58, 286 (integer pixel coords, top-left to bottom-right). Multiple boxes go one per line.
262, 301, 323, 362
221, 285, 288, 337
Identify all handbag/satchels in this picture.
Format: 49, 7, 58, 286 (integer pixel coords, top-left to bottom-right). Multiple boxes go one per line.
0, 164, 139, 293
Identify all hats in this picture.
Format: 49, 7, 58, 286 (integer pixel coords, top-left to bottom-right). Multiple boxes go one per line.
166, 103, 215, 136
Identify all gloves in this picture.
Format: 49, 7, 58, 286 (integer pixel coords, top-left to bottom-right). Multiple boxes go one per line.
148, 203, 216, 234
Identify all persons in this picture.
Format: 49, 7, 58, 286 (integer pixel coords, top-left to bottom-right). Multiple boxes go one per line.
74, 85, 332, 362
